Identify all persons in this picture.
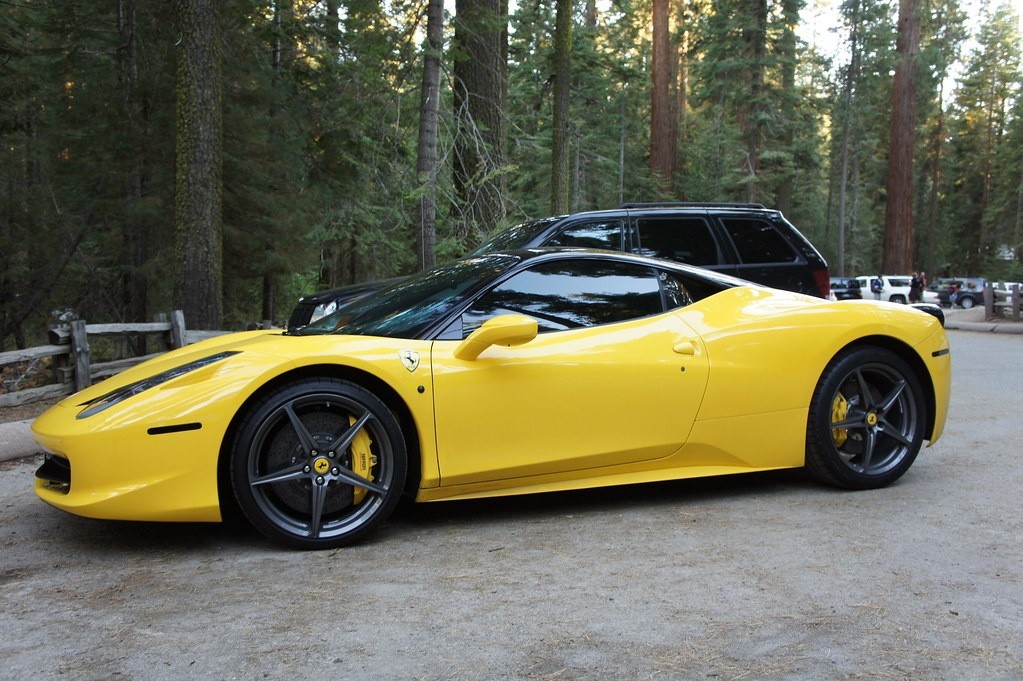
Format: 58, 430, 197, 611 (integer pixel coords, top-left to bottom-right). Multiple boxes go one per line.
909, 272, 926, 303
948, 277, 960, 309
873, 274, 884, 300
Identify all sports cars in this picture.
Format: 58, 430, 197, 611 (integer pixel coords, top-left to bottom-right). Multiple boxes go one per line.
31, 244, 951, 552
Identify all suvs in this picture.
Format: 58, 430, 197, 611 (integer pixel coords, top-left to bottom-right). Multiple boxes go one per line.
926, 277, 986, 309
286, 202, 832, 332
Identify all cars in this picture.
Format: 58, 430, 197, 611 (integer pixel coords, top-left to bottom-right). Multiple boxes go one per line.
988, 281, 1023, 312
828, 275, 942, 307
